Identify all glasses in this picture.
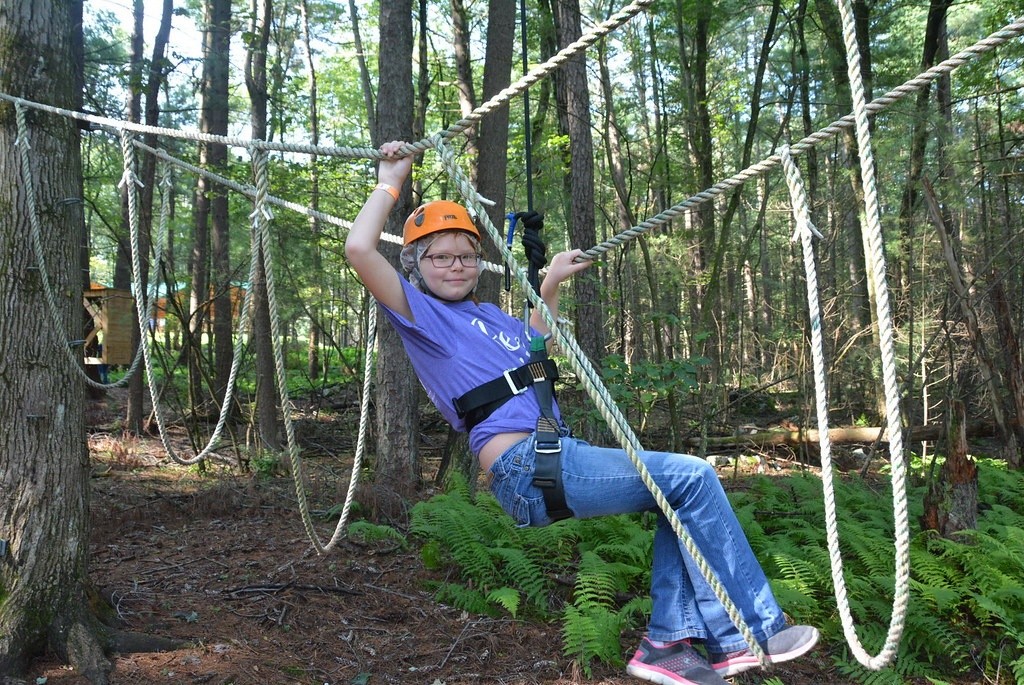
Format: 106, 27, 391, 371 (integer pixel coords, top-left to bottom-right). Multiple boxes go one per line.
421, 252, 483, 269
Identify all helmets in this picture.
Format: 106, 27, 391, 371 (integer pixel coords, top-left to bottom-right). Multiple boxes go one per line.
404, 201, 481, 245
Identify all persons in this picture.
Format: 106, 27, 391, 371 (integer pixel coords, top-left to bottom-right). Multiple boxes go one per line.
345, 141, 819, 685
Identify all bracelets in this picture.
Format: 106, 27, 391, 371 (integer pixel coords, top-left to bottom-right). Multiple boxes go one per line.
375, 183, 399, 201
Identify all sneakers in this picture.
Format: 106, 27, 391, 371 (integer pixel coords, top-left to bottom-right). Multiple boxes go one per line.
711, 625, 820, 679
625, 636, 728, 685
